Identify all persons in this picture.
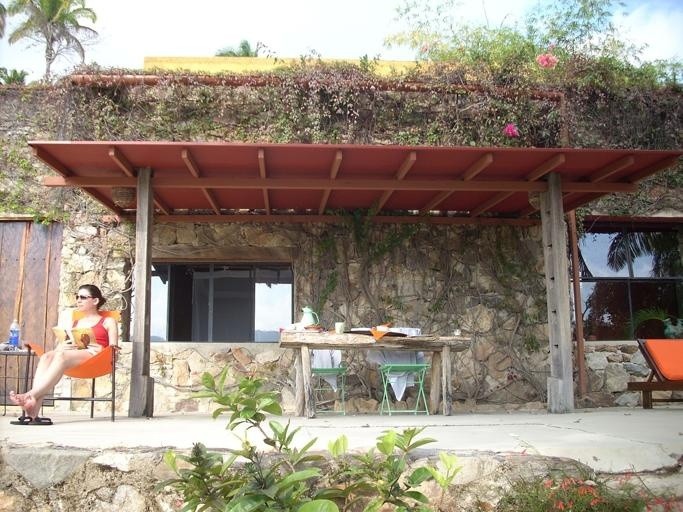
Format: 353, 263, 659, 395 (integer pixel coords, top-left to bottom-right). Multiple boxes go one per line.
9, 284, 118, 421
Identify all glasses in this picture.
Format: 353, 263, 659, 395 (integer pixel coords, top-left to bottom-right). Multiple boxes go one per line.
76, 295, 97, 300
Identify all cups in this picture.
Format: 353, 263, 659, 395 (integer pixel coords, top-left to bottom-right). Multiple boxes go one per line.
335, 322, 345, 334
407, 328, 420, 337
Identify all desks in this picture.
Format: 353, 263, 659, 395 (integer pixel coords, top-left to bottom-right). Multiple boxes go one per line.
0, 350, 43, 415
278, 333, 471, 418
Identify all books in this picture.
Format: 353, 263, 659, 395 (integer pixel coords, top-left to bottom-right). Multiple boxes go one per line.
53, 327, 97, 350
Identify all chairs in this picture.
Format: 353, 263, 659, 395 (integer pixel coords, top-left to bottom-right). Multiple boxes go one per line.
378, 327, 431, 416
22, 311, 121, 421
311, 350, 347, 415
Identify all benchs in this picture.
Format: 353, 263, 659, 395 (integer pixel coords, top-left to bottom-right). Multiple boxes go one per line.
628, 338, 683, 409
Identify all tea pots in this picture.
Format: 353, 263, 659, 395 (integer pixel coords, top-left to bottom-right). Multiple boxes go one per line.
300, 307, 319, 331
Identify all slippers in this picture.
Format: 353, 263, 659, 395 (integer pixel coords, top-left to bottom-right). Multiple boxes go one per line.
10, 416, 53, 425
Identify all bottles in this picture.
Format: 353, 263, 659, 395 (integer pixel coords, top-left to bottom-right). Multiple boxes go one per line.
9, 320, 20, 348
